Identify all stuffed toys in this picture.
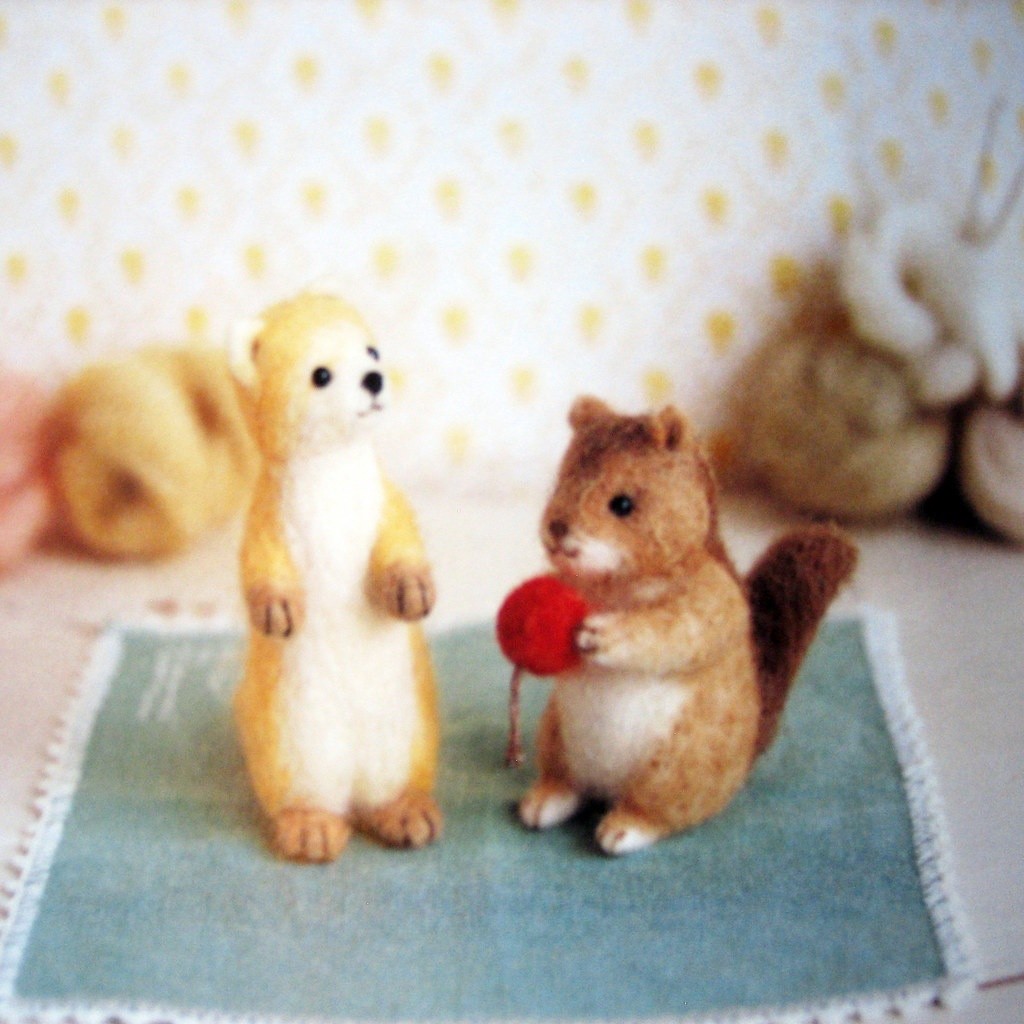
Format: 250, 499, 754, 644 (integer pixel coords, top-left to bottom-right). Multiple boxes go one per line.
495, 398, 861, 855
704, 221, 1024, 537
232, 294, 445, 860
37, 337, 260, 561
